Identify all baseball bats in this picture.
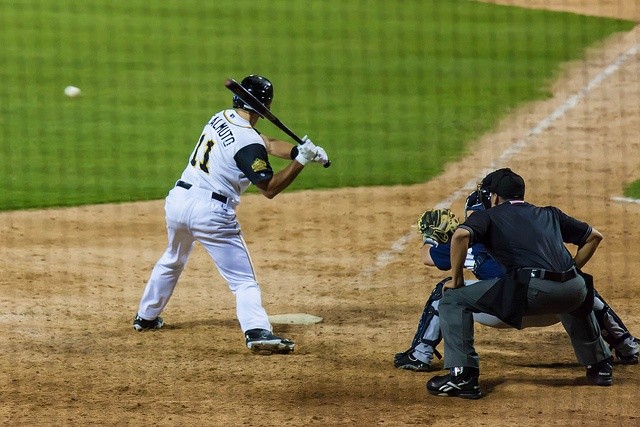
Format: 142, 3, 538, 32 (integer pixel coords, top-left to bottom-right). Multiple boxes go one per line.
224, 77, 331, 169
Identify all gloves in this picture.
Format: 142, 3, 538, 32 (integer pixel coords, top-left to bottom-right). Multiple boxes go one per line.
311, 145, 329, 165
296, 134, 318, 167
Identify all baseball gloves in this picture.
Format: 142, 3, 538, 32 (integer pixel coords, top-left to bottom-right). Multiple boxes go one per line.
418, 208, 458, 243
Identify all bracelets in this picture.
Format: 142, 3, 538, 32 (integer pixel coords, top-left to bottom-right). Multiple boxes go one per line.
289, 145, 298, 160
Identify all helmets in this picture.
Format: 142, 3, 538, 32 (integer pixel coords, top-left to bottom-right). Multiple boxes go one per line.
464, 186, 485, 222
232, 74, 274, 119
477, 166, 525, 208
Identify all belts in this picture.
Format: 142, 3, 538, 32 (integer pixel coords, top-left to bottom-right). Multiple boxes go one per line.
176, 180, 227, 204
530, 268, 577, 284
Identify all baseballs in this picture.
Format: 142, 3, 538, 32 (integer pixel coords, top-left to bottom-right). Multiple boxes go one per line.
65, 86, 80, 97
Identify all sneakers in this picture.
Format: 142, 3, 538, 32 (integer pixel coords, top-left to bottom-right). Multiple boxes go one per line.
426, 371, 482, 400
244, 328, 296, 356
132, 314, 164, 331
394, 352, 431, 372
586, 361, 613, 385
615, 349, 639, 365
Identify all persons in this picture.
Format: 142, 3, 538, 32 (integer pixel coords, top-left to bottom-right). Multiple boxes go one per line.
134, 75, 328, 353
393, 191, 640, 372
426, 168, 613, 400
